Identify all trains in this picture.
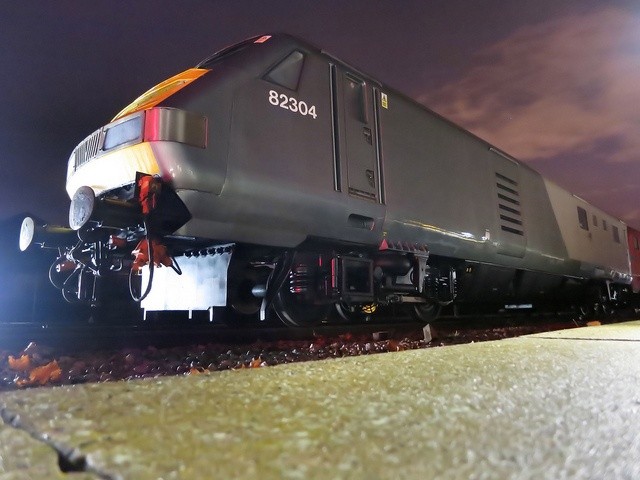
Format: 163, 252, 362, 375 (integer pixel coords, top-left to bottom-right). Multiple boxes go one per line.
12, 32, 635, 332
